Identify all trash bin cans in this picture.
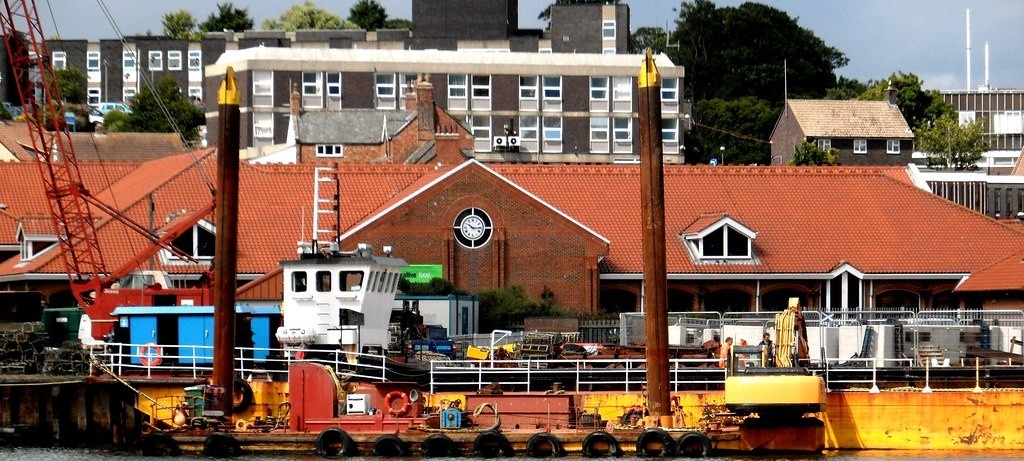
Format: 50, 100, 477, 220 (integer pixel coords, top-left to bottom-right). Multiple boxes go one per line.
727, 345, 766, 376
183, 385, 213, 418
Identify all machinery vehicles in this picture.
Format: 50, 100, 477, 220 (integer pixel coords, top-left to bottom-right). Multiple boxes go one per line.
723, 297, 828, 452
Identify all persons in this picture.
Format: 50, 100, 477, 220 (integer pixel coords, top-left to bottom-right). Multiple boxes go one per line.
759, 333, 775, 368
614, 347, 620, 358
719, 337, 733, 369
736, 336, 751, 370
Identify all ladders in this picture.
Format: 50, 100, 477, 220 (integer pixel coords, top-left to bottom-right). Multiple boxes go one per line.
859, 325, 871, 358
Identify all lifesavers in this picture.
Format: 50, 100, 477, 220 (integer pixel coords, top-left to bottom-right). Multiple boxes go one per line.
203, 432, 242, 457
140, 342, 163, 367
582, 432, 619, 458
233, 379, 253, 414
422, 434, 460, 457
636, 429, 673, 458
385, 390, 409, 416
373, 434, 412, 457
316, 428, 351, 458
474, 431, 515, 458
526, 432, 563, 457
141, 431, 177, 457
674, 431, 712, 457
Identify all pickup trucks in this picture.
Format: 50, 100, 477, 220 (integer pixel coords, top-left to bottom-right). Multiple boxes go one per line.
403, 324, 457, 362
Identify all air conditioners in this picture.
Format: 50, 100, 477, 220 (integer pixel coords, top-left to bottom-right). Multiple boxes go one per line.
508, 136, 520, 146
494, 136, 506, 147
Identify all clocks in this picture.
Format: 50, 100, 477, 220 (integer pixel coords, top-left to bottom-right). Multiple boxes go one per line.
460, 215, 485, 241
